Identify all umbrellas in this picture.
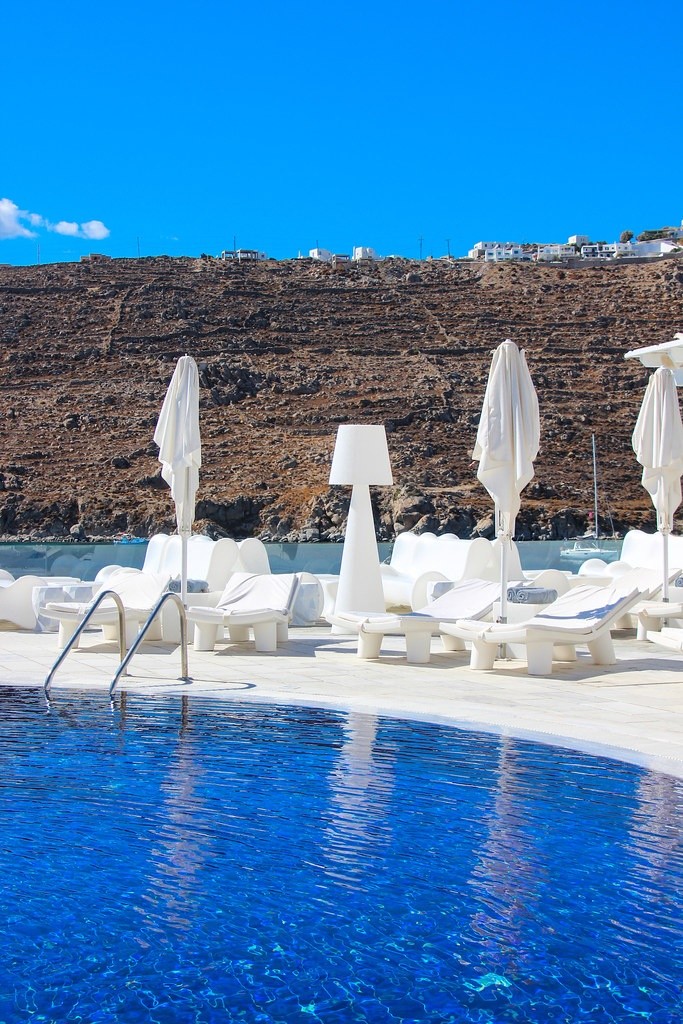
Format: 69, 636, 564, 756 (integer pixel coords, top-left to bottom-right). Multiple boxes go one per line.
151, 352, 212, 612
629, 365, 683, 602
470, 336, 540, 658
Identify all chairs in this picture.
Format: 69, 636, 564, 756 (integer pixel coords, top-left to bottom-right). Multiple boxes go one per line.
0, 530, 683, 676
